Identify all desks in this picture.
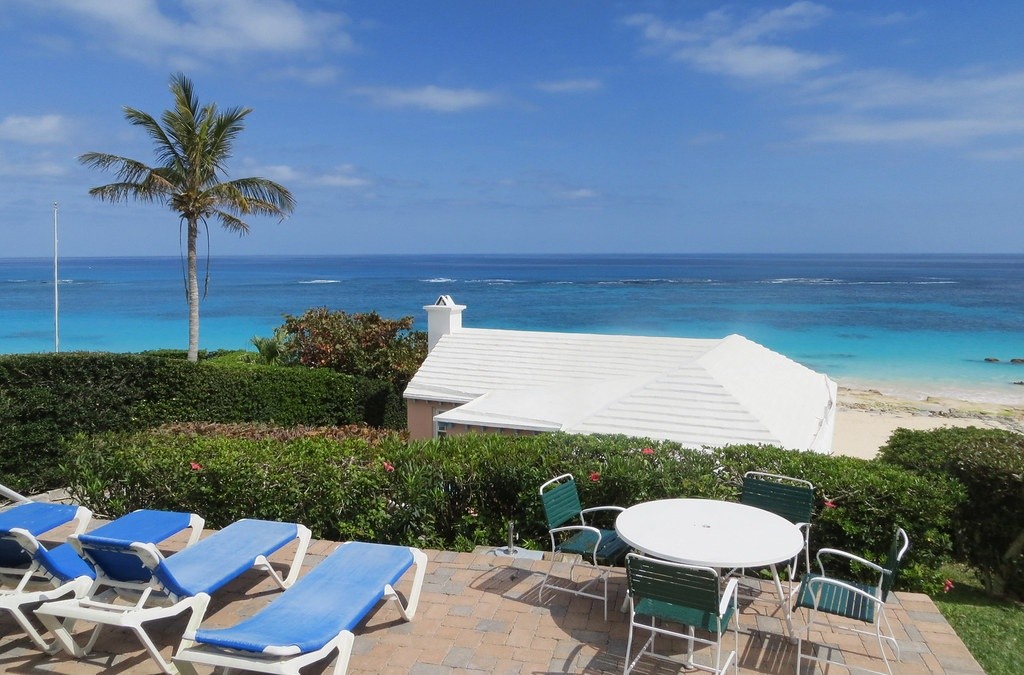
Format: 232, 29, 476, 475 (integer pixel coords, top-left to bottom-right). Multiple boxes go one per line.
615, 498, 804, 645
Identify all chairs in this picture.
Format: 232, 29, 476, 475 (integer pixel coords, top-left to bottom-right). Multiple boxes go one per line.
538, 474, 630, 623
0, 508, 205, 656
793, 528, 909, 675
740, 471, 814, 617
0, 502, 93, 598
170, 540, 428, 675
34, 519, 312, 675
622, 553, 740, 675
